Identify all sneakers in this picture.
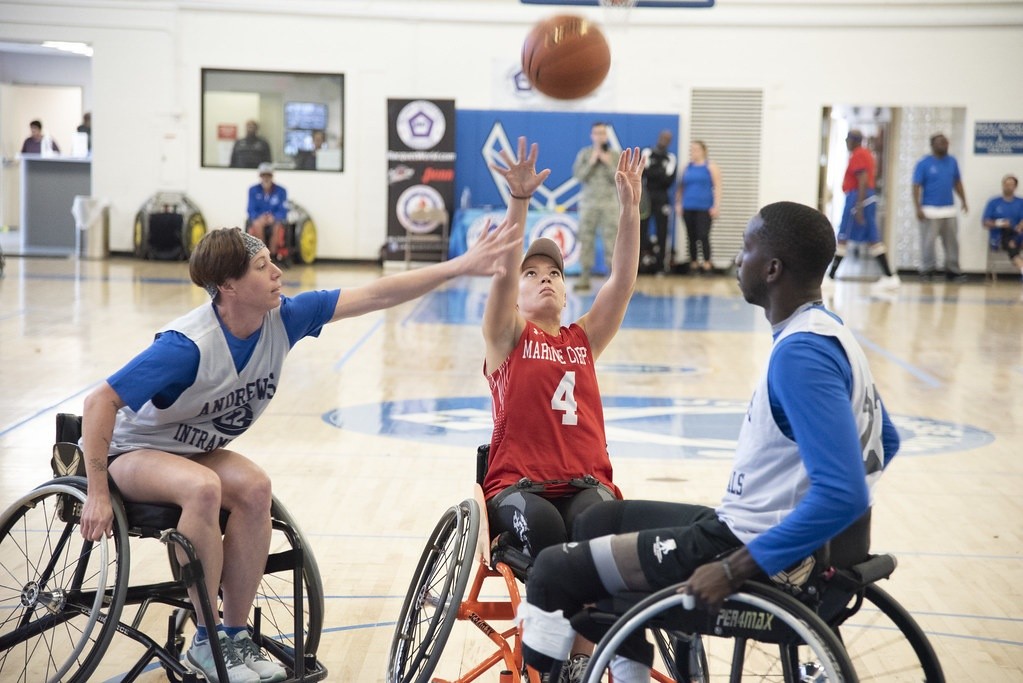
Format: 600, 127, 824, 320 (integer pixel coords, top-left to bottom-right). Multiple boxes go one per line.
570, 658, 589, 683
184, 631, 262, 683
558, 660, 573, 683
231, 630, 287, 683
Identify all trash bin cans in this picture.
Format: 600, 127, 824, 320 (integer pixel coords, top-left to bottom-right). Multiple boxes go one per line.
75, 198, 109, 261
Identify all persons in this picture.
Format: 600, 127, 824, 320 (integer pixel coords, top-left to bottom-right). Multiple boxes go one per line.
296, 130, 323, 169
639, 125, 678, 274
76, 113, 90, 152
677, 140, 719, 276
228, 121, 272, 170
820, 130, 902, 289
571, 122, 623, 292
21, 120, 59, 154
245, 162, 287, 259
476, 134, 656, 683
912, 133, 969, 281
81, 218, 524, 683
518, 203, 901, 683
980, 176, 1023, 275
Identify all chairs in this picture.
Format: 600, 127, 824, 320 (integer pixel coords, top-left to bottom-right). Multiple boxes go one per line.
984, 227, 1023, 283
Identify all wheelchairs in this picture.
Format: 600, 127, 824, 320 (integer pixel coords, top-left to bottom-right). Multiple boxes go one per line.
0, 413, 328, 683
549, 507, 945, 683
387, 446, 709, 683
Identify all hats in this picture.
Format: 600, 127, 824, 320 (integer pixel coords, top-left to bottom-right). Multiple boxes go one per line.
520, 237, 565, 280
257, 162, 273, 177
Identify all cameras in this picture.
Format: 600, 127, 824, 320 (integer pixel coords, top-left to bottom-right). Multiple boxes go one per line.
602, 143, 608, 151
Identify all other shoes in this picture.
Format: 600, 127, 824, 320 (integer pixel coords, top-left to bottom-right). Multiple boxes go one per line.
573, 282, 590, 290
945, 272, 970, 282
873, 274, 900, 289
870, 291, 898, 306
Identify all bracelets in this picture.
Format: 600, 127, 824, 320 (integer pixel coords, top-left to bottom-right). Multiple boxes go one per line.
510, 192, 532, 200
721, 557, 738, 595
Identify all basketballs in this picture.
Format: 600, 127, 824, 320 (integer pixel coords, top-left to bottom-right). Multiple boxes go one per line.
521, 14, 612, 100
